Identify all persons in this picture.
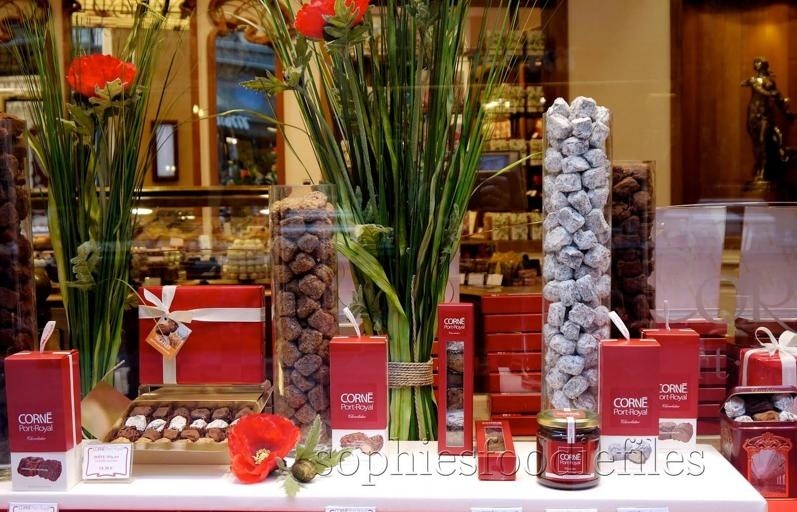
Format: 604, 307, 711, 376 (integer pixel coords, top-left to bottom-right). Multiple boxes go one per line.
738, 56, 778, 181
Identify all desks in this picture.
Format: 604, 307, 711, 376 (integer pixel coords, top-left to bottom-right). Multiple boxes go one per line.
0, 440, 768, 512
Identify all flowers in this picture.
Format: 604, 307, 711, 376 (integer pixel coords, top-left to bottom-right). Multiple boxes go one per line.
0, 0, 205, 440
227, 413, 301, 483
230, 0, 544, 439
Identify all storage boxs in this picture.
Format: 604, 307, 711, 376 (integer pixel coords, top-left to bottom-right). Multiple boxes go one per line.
719, 385, 797, 498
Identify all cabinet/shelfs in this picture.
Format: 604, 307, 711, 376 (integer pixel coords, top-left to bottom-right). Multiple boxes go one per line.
28, 185, 273, 305
317, 0, 570, 295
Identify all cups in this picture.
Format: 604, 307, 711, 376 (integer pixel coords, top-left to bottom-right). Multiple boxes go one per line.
601, 158, 656, 339
265, 180, 340, 454
541, 106, 612, 424
0, 115, 41, 486
